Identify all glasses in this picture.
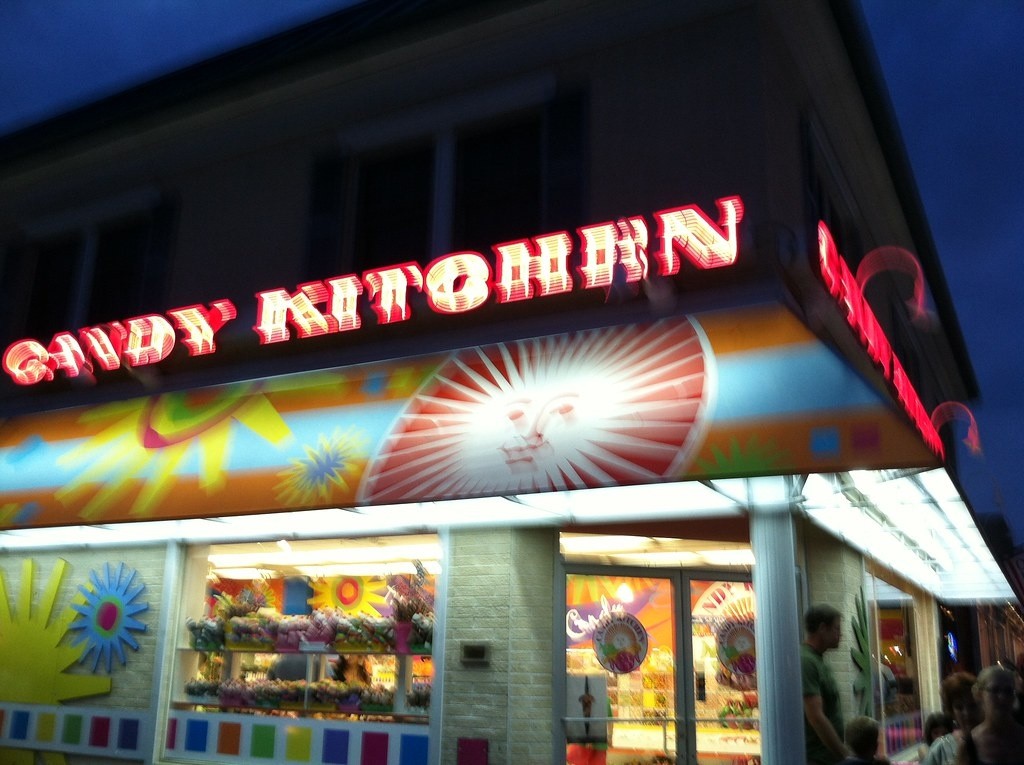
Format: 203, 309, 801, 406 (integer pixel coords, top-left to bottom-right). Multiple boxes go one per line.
979, 687, 1017, 697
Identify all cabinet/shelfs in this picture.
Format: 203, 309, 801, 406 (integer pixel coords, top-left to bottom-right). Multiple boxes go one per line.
174, 646, 431, 723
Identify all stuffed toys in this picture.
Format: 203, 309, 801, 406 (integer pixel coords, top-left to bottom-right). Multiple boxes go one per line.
179, 671, 433, 725
186, 606, 436, 654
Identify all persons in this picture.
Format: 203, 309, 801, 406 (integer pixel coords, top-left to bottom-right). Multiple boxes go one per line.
842, 651, 1024, 765
268, 653, 373, 691
799, 602, 849, 765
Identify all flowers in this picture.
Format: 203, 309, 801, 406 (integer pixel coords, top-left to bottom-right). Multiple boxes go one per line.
183, 601, 433, 648
184, 677, 431, 710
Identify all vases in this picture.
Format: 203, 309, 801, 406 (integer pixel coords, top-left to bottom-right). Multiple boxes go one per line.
395, 621, 412, 653
